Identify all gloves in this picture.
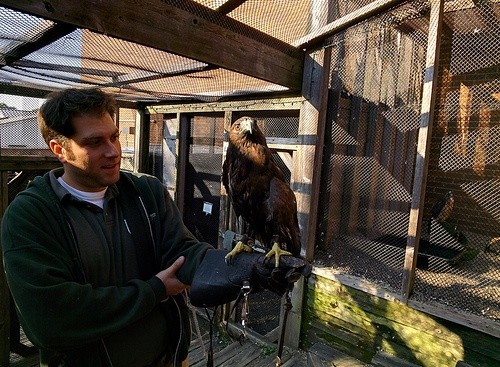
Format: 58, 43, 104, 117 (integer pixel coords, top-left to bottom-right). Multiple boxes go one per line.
188, 246, 313, 309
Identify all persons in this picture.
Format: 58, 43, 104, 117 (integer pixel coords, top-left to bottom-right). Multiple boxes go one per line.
2, 89, 315, 367
